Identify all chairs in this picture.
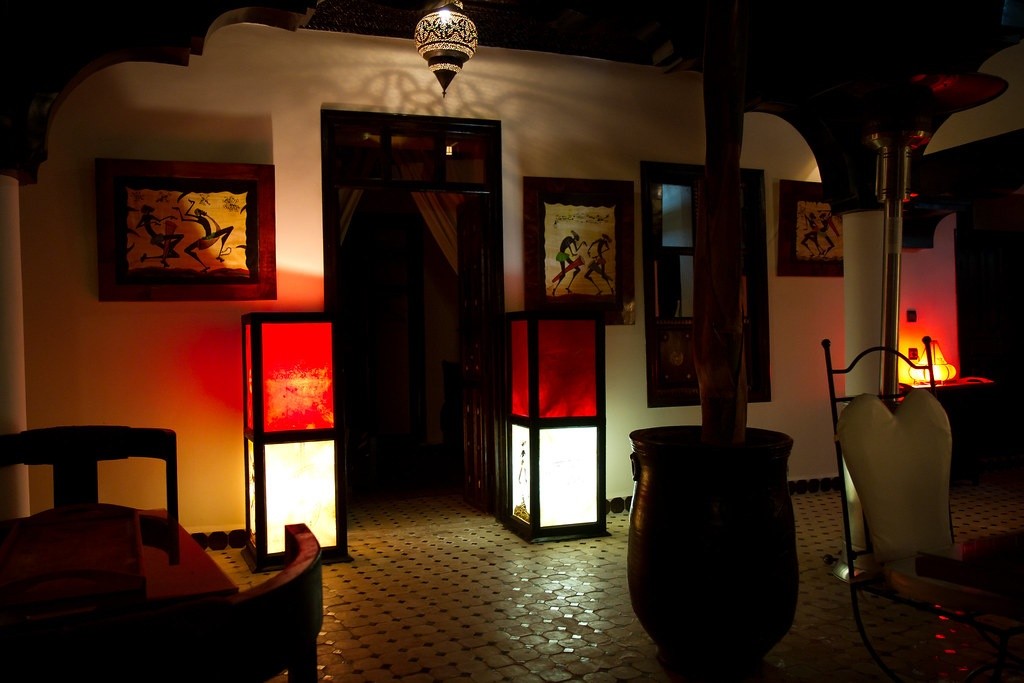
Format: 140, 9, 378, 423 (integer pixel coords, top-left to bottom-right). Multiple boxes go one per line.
0, 524, 334, 683
821, 336, 1023, 683
0, 425, 179, 565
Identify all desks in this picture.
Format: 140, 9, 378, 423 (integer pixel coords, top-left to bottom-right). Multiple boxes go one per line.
0, 508, 240, 621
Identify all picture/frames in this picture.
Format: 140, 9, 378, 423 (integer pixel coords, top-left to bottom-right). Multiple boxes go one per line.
96, 158, 278, 302
777, 179, 845, 277
523, 176, 636, 327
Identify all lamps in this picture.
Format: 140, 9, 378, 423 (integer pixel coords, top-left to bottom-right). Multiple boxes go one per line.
908, 340, 957, 385
503, 311, 613, 544
414, 0, 479, 98
242, 312, 354, 572
